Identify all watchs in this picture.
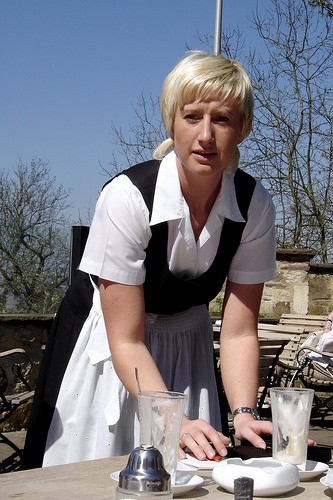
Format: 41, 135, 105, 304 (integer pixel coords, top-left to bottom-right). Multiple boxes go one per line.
232, 407, 261, 421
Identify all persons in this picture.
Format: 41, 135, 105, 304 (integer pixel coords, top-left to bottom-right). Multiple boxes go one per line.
23, 49, 317, 470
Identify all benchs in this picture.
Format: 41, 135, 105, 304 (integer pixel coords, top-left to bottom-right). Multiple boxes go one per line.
0, 313, 333, 474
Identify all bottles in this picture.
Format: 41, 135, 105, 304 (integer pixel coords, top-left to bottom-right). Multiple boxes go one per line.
115, 444, 173, 500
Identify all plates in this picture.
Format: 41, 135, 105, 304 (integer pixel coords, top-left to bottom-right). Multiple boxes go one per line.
109, 469, 204, 496
271, 458, 328, 482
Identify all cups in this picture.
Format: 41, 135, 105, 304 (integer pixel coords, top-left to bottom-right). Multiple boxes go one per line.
269, 386, 314, 471
138, 390, 187, 486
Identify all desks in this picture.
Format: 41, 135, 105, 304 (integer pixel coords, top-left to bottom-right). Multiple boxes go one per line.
0, 435, 333, 500
